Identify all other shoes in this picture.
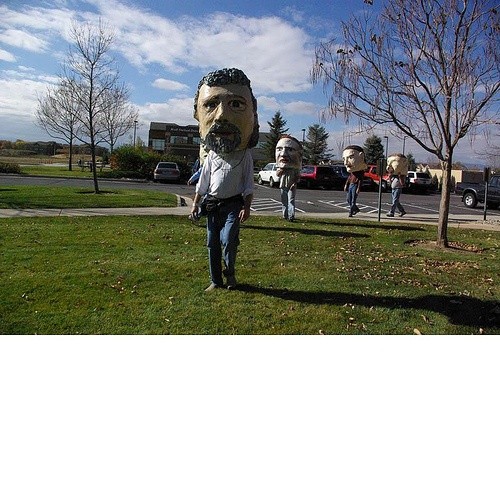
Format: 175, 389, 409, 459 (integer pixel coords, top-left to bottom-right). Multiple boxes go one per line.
349, 213, 352, 217
226, 277, 237, 290
288, 217, 293, 222
387, 213, 394, 217
398, 211, 406, 216
204, 280, 223, 291
352, 209, 360, 215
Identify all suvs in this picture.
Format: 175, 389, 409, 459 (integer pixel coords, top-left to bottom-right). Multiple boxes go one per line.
190, 158, 201, 184
403, 171, 434, 194
258, 163, 281, 187
297, 165, 332, 189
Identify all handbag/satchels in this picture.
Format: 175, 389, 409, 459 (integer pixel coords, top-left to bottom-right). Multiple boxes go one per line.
200, 197, 225, 216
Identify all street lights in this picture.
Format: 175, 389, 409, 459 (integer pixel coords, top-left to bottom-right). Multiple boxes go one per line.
133, 120, 138, 147
383, 136, 389, 161
302, 128, 306, 142
403, 136, 408, 156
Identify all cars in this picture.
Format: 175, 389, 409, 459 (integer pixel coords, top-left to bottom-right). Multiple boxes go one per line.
153, 162, 181, 185
253, 167, 262, 181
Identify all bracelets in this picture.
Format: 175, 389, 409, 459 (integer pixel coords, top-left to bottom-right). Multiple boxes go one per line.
193, 203, 198, 207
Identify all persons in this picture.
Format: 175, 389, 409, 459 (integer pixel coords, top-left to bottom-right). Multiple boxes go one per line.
275, 135, 302, 221
191, 68, 260, 291
188, 143, 211, 223
386, 153, 408, 217
342, 145, 368, 217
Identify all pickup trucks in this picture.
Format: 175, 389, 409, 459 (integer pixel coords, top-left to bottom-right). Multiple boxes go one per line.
456, 175, 500, 211
320, 165, 372, 192
364, 165, 391, 192
